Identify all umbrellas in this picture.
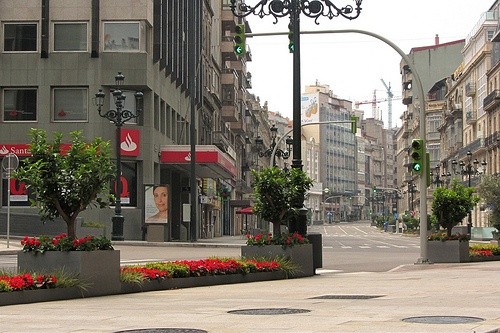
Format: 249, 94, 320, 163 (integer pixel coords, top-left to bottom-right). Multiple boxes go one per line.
237, 207, 257, 215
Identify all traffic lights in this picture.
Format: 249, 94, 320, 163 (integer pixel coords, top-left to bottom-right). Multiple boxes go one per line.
234, 24, 245, 55
373, 189, 377, 193
412, 139, 424, 175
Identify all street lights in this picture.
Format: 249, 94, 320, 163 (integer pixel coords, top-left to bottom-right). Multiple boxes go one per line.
374, 186, 402, 223
95, 69, 144, 240
229, 0, 365, 236
430, 165, 451, 231
452, 150, 488, 234
408, 183, 416, 211
255, 124, 292, 238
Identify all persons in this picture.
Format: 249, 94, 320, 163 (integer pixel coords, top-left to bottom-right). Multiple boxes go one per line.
146, 186, 168, 221
